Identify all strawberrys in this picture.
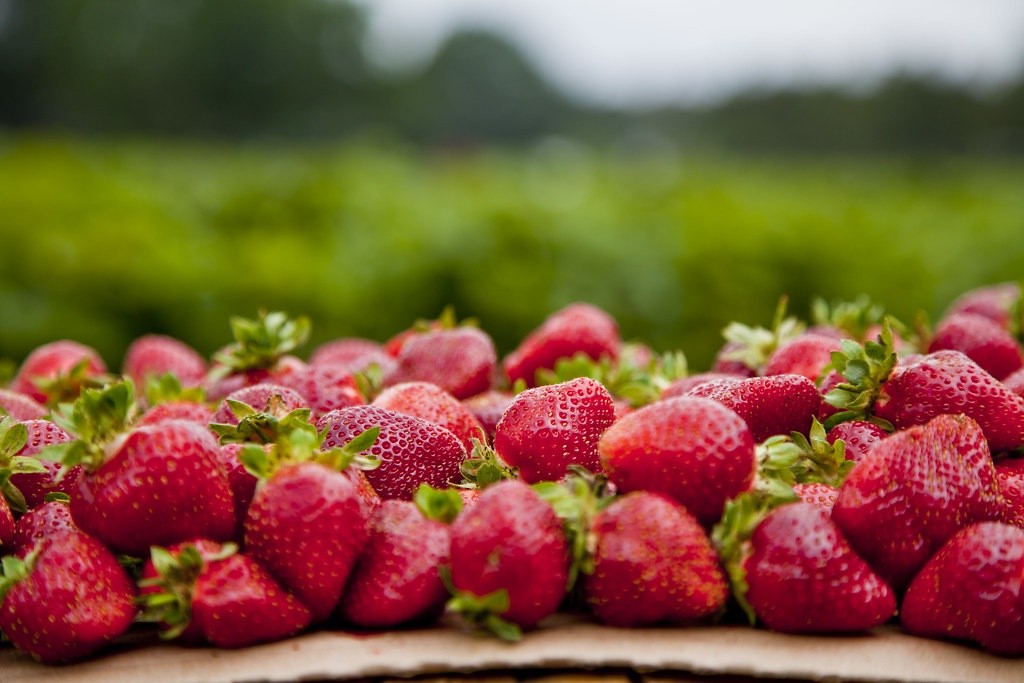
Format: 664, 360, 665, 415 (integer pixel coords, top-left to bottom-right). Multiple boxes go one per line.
0, 284, 1024, 663
